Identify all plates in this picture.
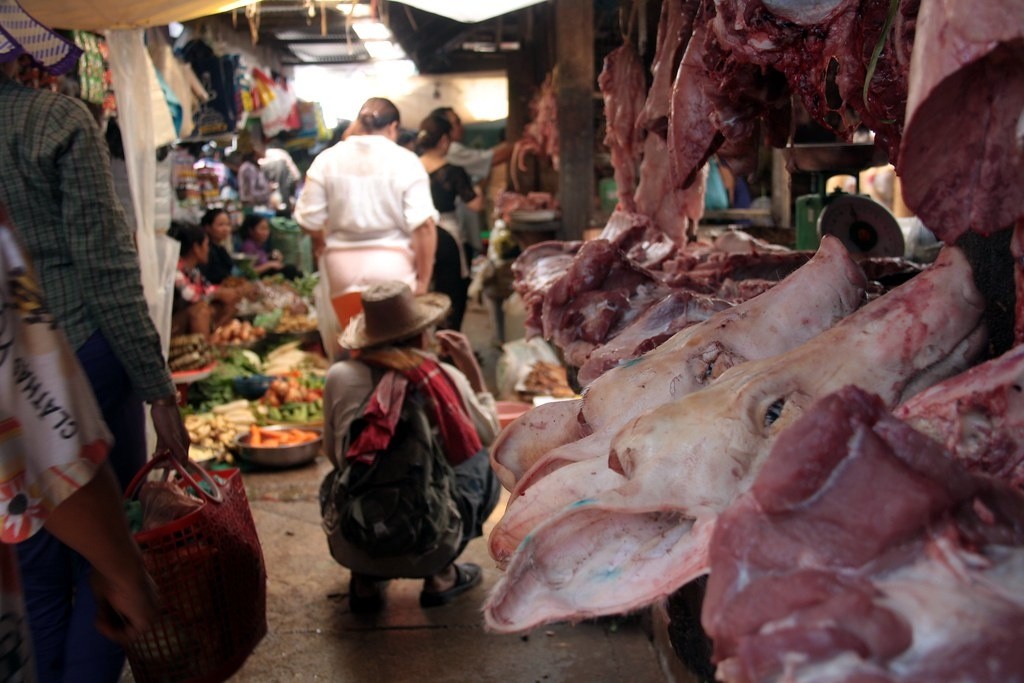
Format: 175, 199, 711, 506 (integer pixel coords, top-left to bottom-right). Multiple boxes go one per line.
510, 209, 556, 221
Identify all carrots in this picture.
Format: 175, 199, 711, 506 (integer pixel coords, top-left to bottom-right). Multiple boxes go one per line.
211, 319, 264, 346
245, 424, 319, 447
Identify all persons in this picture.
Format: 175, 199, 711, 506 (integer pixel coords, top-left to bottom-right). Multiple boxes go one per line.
327, 114, 511, 221
317, 280, 502, 618
296, 97, 440, 362
1, 184, 166, 683
1, 53, 192, 683
402, 114, 487, 368
155, 131, 303, 346
429, 106, 516, 332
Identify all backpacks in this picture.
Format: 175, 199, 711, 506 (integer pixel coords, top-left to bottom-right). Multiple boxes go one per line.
335, 363, 452, 559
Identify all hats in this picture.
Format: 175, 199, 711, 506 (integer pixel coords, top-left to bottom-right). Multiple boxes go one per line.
337, 279, 451, 349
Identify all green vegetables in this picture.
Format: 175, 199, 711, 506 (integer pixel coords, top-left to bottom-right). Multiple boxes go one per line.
176, 347, 267, 416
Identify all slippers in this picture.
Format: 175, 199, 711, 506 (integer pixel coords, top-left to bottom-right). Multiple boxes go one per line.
420, 562, 482, 604
349, 577, 392, 620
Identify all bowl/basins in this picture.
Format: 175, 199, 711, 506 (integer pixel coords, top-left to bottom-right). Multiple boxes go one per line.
232, 424, 326, 470
495, 401, 534, 429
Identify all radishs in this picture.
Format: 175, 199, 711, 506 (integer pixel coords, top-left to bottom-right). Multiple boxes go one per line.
212, 398, 257, 433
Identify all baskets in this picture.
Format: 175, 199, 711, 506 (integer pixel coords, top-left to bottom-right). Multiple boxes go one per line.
120, 450, 268, 683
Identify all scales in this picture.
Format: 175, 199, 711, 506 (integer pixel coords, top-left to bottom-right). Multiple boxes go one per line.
229, 252, 260, 281
788, 140, 907, 264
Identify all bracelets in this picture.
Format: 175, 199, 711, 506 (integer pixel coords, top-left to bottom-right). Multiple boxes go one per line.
150, 389, 184, 407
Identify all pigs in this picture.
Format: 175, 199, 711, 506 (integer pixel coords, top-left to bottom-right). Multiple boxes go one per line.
481, 229, 993, 638
